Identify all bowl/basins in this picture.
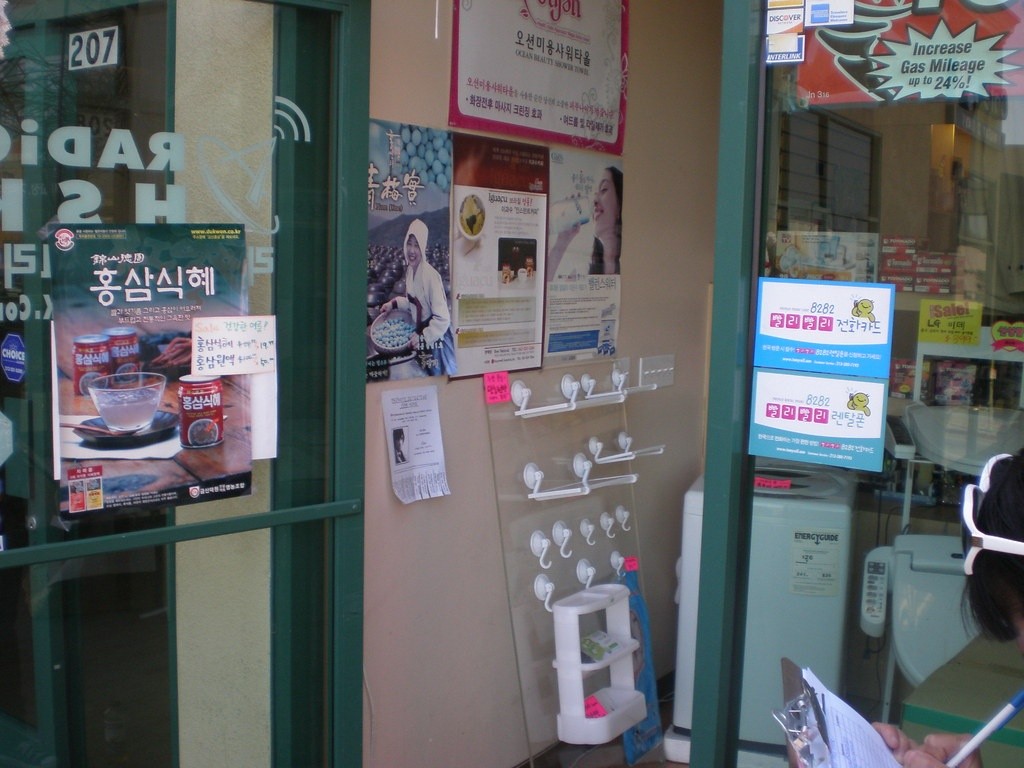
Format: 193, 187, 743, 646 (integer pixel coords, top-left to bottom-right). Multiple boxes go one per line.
88, 372, 166, 433
457, 193, 487, 241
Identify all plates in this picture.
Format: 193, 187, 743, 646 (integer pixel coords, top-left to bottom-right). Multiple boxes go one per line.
73, 411, 179, 443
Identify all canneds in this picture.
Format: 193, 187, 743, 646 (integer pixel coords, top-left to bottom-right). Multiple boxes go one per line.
73, 327, 227, 449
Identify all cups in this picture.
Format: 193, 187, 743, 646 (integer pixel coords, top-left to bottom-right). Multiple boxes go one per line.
548, 196, 592, 235
518, 268, 527, 280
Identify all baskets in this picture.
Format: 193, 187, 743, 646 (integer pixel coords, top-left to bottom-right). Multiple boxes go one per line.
370, 308, 416, 354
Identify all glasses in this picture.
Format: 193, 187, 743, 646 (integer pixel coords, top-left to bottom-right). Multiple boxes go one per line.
961, 451, 1024, 575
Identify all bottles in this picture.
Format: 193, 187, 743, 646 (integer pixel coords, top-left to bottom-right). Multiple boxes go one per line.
525, 256, 533, 278
502, 264, 511, 284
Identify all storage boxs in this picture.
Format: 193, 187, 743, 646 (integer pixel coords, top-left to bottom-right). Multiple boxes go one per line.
915, 252, 957, 275
888, 357, 933, 399
880, 254, 916, 275
773, 231, 879, 283
880, 236, 928, 253
881, 275, 914, 292
933, 361, 977, 402
913, 275, 951, 293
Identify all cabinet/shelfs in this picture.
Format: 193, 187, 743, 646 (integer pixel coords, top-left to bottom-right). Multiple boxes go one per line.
868, 291, 1021, 505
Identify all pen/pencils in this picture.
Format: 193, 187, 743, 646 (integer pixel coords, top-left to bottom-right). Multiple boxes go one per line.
946, 687, 1024, 768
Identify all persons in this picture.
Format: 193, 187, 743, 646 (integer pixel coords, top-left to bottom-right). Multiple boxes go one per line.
546, 166, 624, 282
393, 429, 406, 463
786, 445, 1024, 768
380, 218, 457, 376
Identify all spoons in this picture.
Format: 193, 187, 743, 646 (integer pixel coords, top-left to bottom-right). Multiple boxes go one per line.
60, 423, 136, 436
